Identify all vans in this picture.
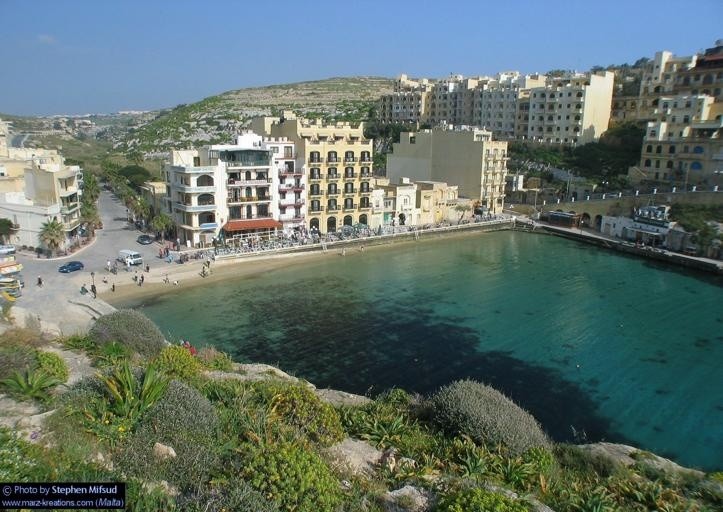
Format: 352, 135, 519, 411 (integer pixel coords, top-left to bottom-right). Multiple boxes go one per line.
0, 245, 16, 255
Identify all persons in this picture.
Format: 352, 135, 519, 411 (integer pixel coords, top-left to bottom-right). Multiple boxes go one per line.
211, 253, 215, 262
80, 283, 88, 295
212, 235, 217, 247
206, 256, 210, 268
159, 237, 180, 259
103, 277, 108, 283
113, 261, 118, 275
165, 274, 169, 283
134, 269, 145, 286
112, 283, 115, 291
93, 286, 96, 298
106, 258, 111, 272
37, 276, 42, 288
145, 263, 150, 272
173, 280, 179, 285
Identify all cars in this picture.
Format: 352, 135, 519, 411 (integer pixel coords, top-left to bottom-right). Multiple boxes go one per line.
138, 235, 152, 245
59, 261, 84, 273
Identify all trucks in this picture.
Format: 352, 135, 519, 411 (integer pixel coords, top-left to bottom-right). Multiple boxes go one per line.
119, 249, 142, 265
